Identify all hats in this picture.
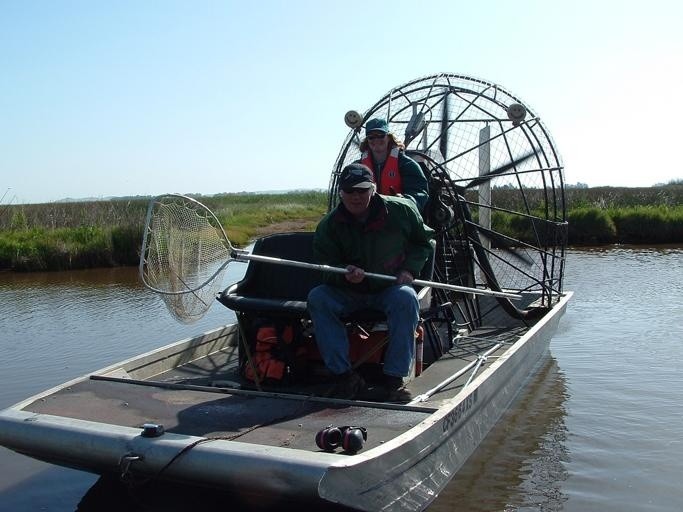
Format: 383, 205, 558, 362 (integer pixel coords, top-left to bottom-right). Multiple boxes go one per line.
338, 164, 374, 190
365, 118, 389, 136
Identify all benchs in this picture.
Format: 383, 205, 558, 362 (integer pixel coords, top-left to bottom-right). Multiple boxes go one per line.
216, 232, 438, 393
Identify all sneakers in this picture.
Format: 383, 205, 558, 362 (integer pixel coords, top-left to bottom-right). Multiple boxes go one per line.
326, 369, 366, 409
378, 372, 412, 403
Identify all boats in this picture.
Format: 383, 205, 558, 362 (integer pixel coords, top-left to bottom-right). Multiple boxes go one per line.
326, 338, 563, 510
0, 75, 570, 511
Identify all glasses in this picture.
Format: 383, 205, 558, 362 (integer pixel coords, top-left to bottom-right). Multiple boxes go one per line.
366, 133, 388, 139
342, 189, 370, 194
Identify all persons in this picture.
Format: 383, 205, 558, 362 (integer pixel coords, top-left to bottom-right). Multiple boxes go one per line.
348, 118, 430, 217
304, 163, 434, 408
